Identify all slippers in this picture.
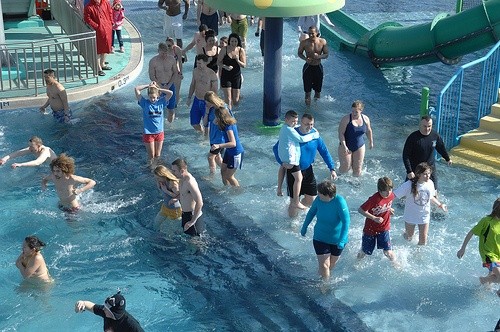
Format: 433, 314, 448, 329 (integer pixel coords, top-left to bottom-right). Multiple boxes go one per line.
98, 71, 106, 76
102, 66, 112, 70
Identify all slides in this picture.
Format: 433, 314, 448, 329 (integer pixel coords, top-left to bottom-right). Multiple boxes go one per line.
319, 0, 500, 70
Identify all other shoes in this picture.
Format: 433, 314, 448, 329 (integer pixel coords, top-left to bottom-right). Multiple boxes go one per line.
111, 46, 114, 53
120, 46, 125, 53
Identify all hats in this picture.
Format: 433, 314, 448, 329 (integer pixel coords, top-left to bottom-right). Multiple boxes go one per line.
111, 0, 124, 10
104, 291, 126, 321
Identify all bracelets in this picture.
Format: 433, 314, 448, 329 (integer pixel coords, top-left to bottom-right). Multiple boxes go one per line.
330, 168, 336, 171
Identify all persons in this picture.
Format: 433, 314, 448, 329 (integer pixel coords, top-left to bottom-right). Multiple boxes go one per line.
135, 81, 173, 160
39, 69, 73, 122
457, 198, 500, 284
337, 100, 373, 176
298, 26, 328, 107
358, 177, 397, 260
402, 115, 452, 189
109, 0, 126, 53
0, 136, 58, 168
84, 0, 115, 76
273, 110, 337, 216
203, 91, 224, 167
15, 235, 48, 279
211, 107, 244, 187
75, 291, 146, 332
153, 165, 182, 220
301, 181, 350, 276
172, 158, 204, 237
148, 0, 265, 131
393, 163, 447, 245
42, 155, 96, 212
